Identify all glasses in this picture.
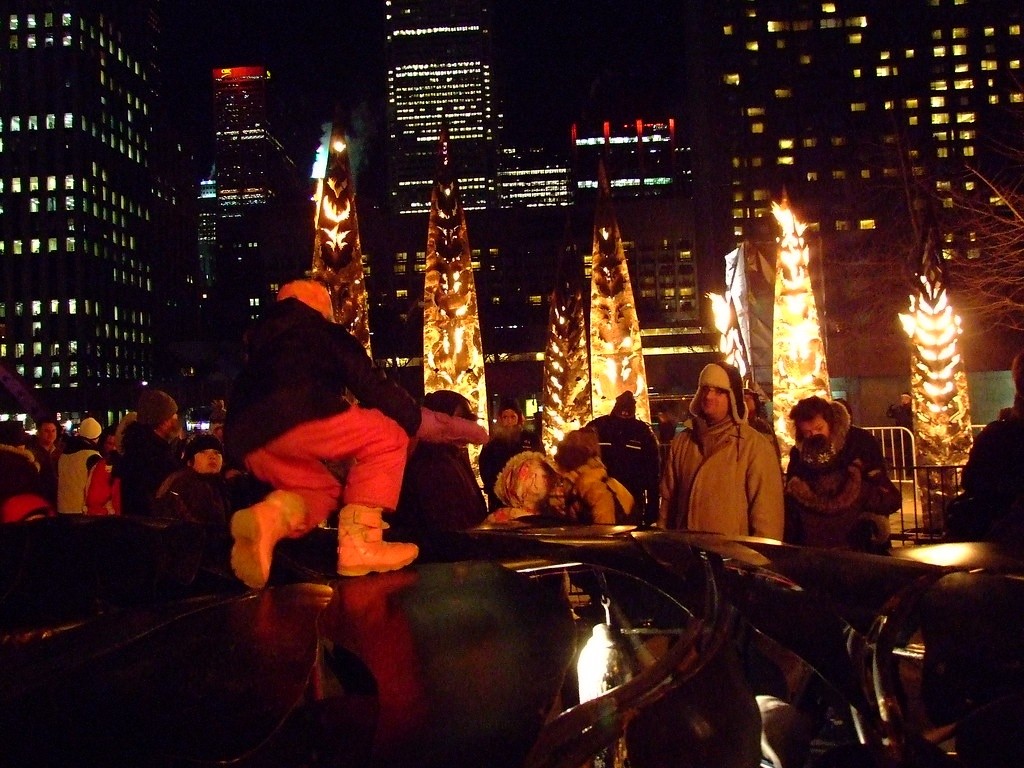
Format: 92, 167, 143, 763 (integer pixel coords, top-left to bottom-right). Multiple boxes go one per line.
454, 412, 478, 422
700, 386, 726, 396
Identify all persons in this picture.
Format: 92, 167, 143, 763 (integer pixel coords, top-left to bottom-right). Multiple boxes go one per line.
3, 268, 1023, 652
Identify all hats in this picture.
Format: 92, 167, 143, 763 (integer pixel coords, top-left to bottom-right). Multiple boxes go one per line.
136, 389, 178, 429
184, 435, 225, 461
689, 361, 748, 425
616, 391, 636, 406
77, 417, 103, 440
2, 495, 56, 523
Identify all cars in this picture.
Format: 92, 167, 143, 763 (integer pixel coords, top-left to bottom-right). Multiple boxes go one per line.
1, 494, 1023, 768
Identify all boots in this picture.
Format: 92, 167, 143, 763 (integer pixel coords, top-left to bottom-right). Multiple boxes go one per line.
337, 505, 419, 577
228, 488, 305, 589
